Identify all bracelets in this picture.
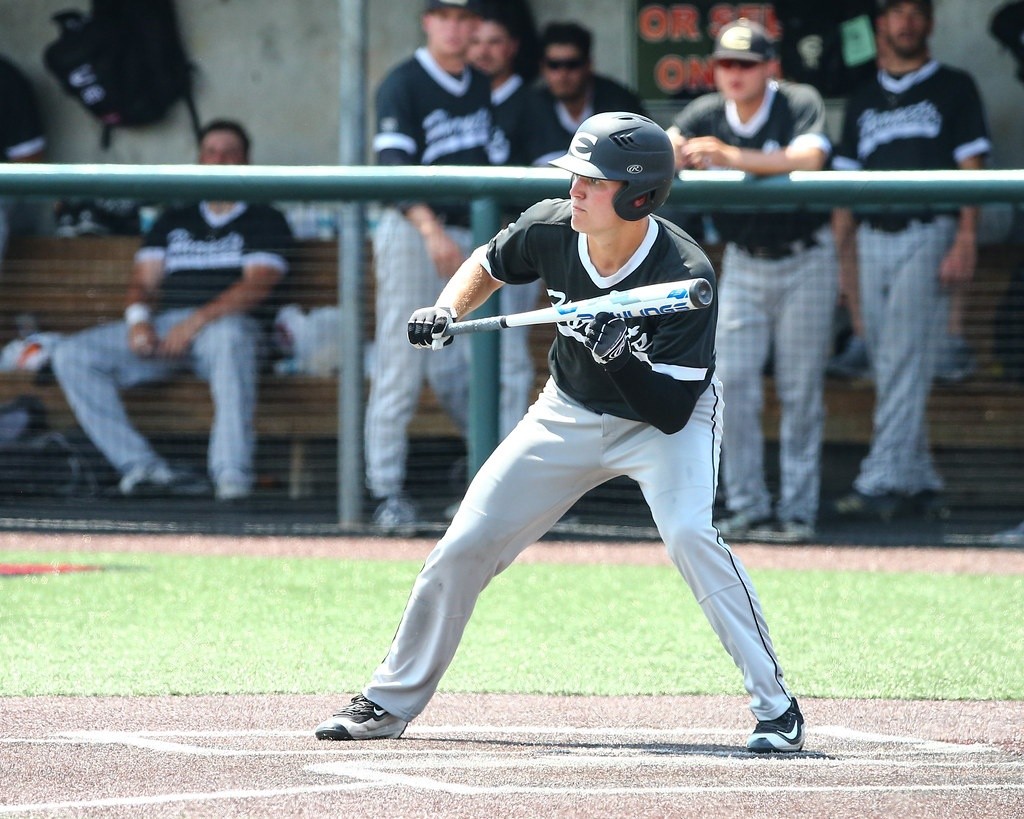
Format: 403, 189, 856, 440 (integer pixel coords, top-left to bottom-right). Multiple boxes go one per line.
123, 302, 151, 324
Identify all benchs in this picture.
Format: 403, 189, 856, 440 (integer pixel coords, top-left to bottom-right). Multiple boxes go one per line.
1, 241, 1024, 496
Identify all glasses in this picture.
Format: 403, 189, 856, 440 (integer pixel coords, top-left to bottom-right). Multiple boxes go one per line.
546, 58, 585, 71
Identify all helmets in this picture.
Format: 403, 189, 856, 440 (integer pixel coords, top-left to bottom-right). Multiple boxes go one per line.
547, 112, 677, 223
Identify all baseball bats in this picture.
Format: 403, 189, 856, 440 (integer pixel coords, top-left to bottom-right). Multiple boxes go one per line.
441, 277, 714, 338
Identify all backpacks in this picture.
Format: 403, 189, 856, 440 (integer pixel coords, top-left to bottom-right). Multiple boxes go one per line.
41, 0, 199, 136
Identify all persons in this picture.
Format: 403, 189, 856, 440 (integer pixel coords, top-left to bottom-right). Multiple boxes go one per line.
314, 112, 805, 753
51, 120, 292, 501
365, 0, 994, 543
0, 54, 52, 258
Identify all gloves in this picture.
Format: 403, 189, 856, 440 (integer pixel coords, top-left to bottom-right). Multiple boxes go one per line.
407, 306, 458, 351
584, 312, 627, 364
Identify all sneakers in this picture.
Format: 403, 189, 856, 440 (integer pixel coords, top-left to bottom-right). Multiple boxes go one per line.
747, 697, 805, 753
315, 694, 409, 740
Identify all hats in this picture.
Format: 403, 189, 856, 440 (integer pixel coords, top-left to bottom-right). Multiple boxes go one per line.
425, 0, 485, 19
712, 17, 779, 64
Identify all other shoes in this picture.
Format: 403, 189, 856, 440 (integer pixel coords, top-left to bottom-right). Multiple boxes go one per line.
782, 513, 817, 543
915, 489, 951, 527
820, 489, 900, 522
119, 464, 183, 497
214, 466, 255, 502
714, 502, 770, 534
374, 497, 419, 537
